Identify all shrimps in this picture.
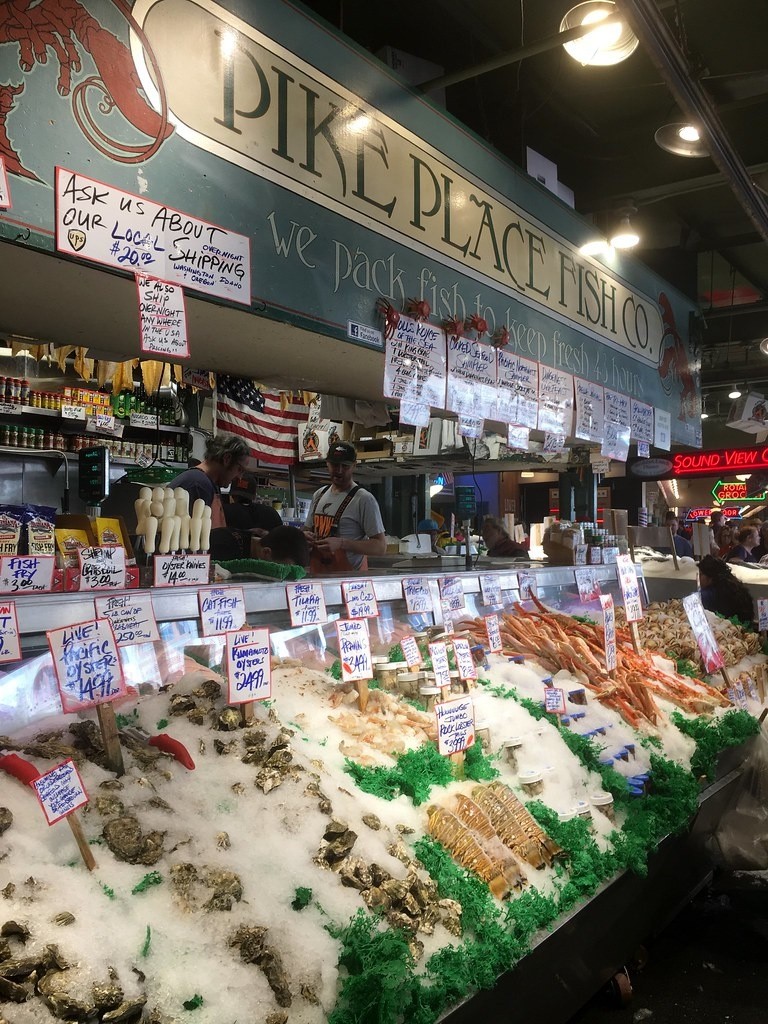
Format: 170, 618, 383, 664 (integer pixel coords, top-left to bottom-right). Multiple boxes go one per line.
328, 677, 441, 769
425, 781, 573, 907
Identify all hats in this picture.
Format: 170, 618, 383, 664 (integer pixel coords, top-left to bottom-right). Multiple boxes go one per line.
417, 519, 440, 531
749, 518, 762, 524
327, 440, 357, 466
229, 474, 257, 500
484, 517, 510, 535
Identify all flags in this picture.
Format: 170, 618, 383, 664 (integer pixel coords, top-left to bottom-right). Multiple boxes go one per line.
213, 373, 310, 465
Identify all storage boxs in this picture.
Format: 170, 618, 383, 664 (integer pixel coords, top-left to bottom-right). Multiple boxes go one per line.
352, 429, 415, 459
724, 391, 768, 443
0, 513, 140, 597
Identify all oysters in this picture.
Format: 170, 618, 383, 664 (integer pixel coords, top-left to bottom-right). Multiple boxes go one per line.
0, 675, 464, 1024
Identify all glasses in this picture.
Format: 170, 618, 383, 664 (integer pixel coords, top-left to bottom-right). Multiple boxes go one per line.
237, 463, 246, 476
721, 534, 731, 538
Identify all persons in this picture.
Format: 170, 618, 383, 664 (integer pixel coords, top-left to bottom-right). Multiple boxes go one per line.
223, 472, 282, 537
698, 511, 768, 569
209, 525, 307, 565
655, 512, 692, 556
482, 517, 529, 557
302, 440, 387, 571
697, 555, 755, 623
166, 435, 249, 516
416, 519, 447, 556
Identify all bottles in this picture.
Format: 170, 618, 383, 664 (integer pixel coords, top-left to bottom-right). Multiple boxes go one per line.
0, 376, 176, 453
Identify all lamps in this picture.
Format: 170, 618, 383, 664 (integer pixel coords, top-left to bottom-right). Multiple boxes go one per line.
610, 198, 639, 248
559, 0, 639, 67
654, 103, 712, 158
728, 384, 741, 399
701, 393, 710, 418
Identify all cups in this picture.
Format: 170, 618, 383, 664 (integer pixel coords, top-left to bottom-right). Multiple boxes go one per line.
272, 500, 282, 510
285, 508, 294, 518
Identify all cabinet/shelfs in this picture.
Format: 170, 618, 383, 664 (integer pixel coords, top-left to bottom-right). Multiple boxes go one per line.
0, 402, 190, 470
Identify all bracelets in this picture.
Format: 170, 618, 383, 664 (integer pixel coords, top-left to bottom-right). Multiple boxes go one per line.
341, 538, 343, 549
